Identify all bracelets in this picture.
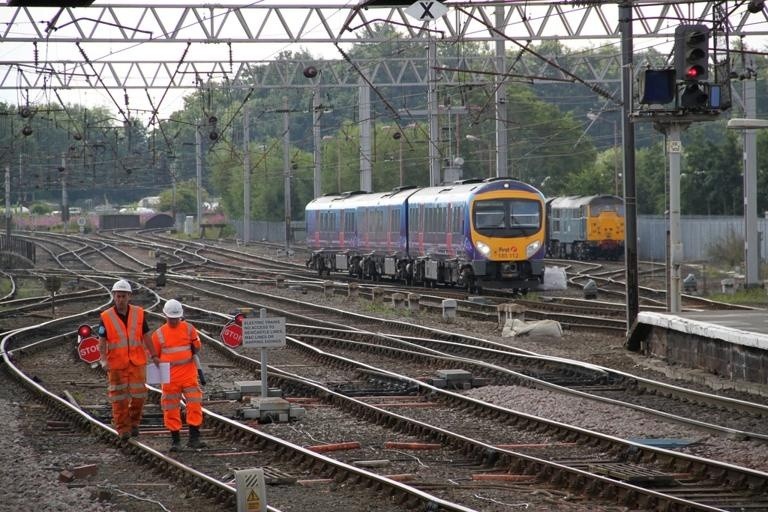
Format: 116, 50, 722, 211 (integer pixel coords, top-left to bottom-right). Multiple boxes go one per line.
150, 354, 159, 359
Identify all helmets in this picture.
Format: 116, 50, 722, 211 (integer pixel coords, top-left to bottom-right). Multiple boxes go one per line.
111, 280, 133, 293
163, 298, 183, 319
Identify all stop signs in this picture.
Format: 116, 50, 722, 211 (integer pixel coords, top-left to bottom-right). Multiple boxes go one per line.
76, 336, 102, 364
221, 322, 244, 350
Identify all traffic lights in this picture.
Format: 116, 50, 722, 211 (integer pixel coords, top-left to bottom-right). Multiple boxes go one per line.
675, 24, 709, 83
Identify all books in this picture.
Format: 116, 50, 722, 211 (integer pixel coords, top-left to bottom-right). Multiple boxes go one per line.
144, 362, 171, 384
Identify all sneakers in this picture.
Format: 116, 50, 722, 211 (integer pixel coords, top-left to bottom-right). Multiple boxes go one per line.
169, 439, 205, 452
121, 425, 138, 441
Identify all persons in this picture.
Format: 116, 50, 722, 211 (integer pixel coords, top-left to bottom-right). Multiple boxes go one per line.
98, 278, 161, 447
147, 297, 213, 451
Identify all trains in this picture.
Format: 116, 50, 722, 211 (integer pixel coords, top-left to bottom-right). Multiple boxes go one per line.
306, 176, 548, 294
544, 194, 626, 262
